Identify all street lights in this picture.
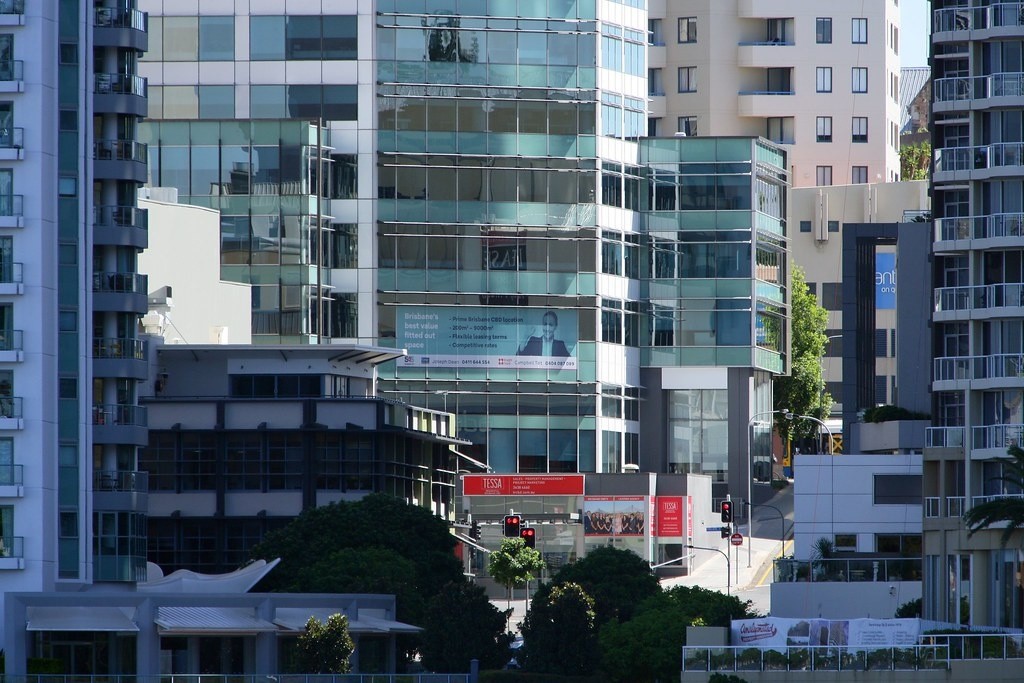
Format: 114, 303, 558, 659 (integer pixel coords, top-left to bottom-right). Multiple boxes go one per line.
785, 413, 834, 456
744, 500, 785, 559
747, 408, 788, 568
684, 545, 730, 597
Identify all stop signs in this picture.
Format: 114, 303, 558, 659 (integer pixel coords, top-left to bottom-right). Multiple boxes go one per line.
732, 534, 743, 546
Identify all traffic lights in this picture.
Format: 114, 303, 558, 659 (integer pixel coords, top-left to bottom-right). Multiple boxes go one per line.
720, 500, 732, 523
519, 528, 536, 550
502, 515, 521, 537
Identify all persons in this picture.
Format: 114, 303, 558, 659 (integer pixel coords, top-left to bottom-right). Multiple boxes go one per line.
584, 511, 613, 534
620, 512, 644, 534
516, 311, 572, 357
